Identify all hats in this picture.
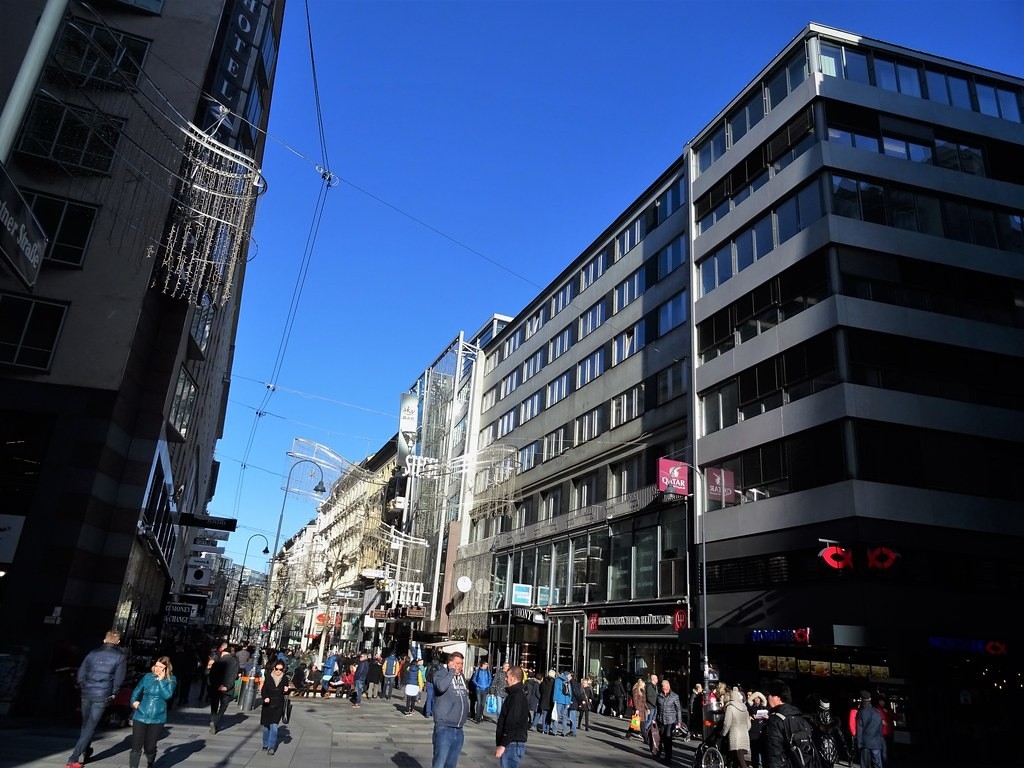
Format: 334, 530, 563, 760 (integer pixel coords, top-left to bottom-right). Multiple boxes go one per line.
819, 698, 831, 710
762, 679, 791, 696
731, 687, 744, 701
222, 647, 232, 653
859, 690, 872, 699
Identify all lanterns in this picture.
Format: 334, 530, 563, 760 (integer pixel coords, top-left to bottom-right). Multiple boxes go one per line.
318, 613, 331, 622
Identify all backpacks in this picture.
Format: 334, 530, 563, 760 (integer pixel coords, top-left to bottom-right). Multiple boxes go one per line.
771, 712, 822, 768
558, 677, 569, 696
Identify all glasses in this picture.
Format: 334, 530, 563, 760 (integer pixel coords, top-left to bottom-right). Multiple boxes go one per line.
275, 666, 283, 672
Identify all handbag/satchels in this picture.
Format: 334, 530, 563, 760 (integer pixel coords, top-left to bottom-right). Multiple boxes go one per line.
282, 691, 292, 724
629, 712, 641, 732
650, 722, 661, 755
550, 702, 558, 721
486, 694, 498, 713
415, 691, 421, 703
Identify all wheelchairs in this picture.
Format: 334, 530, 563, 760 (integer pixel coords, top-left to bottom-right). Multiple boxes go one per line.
692, 733, 725, 768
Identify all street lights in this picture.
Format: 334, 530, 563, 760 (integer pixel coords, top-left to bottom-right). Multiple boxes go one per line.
488, 533, 515, 664
241, 459, 329, 713
226, 533, 271, 640
661, 464, 711, 706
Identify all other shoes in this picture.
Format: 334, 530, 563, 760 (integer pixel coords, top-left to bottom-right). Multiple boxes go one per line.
65, 761, 84, 768
643, 739, 650, 745
659, 757, 672, 766
622, 736, 631, 740
261, 746, 268, 752
268, 748, 275, 755
690, 735, 700, 740
209, 722, 215, 735
83, 748, 93, 762
652, 752, 660, 759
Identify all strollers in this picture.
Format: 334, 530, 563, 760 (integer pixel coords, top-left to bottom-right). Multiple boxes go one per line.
672, 720, 694, 743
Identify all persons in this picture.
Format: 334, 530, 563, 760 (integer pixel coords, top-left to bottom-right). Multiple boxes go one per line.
130, 655, 176, 768
748, 689, 900, 768
493, 662, 593, 738
199, 634, 294, 755
295, 643, 399, 708
496, 666, 529, 768
432, 652, 470, 768
400, 653, 492, 724
64, 629, 126, 768
178, 641, 198, 707
595, 660, 751, 768
